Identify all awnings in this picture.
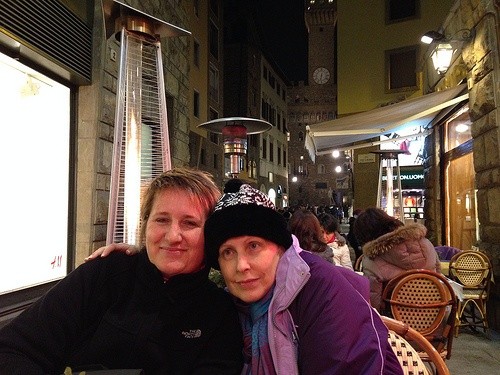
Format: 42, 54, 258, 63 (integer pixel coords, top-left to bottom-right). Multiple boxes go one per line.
304, 85, 469, 163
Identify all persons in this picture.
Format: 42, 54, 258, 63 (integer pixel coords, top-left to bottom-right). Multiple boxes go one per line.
83, 179, 403, 375
0, 168, 244, 375
286, 201, 441, 317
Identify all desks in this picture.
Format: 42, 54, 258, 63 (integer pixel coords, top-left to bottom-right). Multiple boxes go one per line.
440, 262, 495, 333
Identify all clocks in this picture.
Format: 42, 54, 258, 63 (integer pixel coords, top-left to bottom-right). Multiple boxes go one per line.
313, 66, 331, 85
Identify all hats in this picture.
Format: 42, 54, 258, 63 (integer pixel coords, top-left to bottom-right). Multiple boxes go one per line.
204, 178, 293, 271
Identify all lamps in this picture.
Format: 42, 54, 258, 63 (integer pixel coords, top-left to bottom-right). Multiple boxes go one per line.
428, 43, 464, 78
420, 28, 476, 45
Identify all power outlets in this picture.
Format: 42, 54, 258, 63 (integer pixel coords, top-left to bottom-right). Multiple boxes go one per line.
111, 49, 117, 62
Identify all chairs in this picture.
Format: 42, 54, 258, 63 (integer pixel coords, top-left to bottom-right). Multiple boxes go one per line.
448, 249, 493, 338
380, 269, 458, 375
380, 314, 451, 375
435, 246, 465, 261
